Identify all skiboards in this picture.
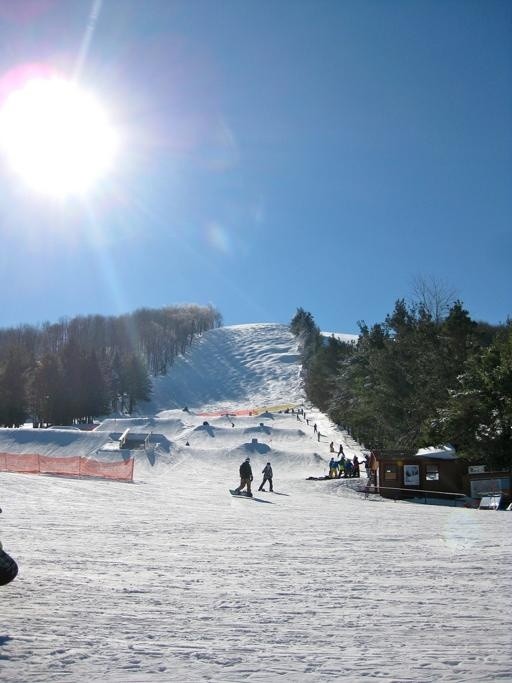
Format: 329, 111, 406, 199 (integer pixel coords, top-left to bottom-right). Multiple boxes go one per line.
259, 489, 279, 494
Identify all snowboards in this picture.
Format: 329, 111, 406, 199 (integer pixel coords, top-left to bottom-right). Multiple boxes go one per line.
229, 489, 252, 496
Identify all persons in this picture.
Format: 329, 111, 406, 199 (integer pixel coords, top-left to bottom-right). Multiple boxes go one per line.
235, 458, 253, 495
329, 454, 369, 478
330, 442, 334, 452
338, 444, 345, 458
314, 424, 317, 433
258, 462, 273, 492
